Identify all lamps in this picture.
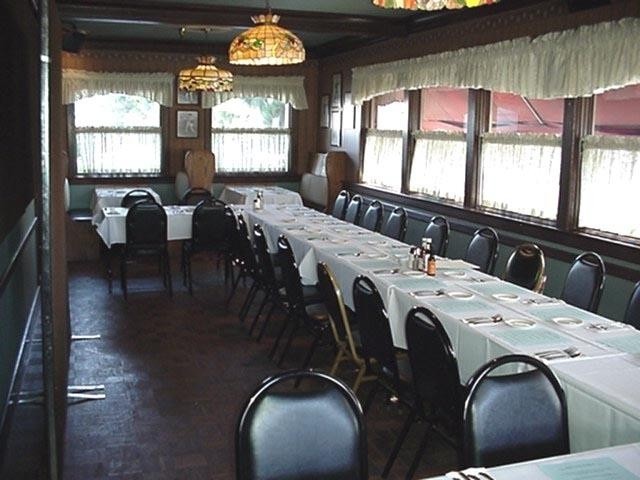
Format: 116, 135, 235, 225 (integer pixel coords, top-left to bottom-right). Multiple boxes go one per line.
228, 13, 307, 66
176, 53, 234, 93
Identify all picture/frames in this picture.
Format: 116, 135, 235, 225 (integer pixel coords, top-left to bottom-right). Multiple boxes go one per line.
176, 109, 200, 139
331, 72, 342, 109
318, 93, 331, 128
344, 91, 355, 130
330, 110, 343, 147
178, 88, 201, 106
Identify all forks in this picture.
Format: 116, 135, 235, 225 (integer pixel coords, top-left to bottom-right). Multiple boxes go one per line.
540, 347, 578, 358
535, 346, 573, 356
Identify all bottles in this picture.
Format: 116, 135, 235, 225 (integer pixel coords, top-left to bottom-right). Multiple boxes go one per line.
427, 246, 436, 276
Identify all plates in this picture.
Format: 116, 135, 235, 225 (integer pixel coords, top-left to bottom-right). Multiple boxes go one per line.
404, 271, 427, 279
448, 291, 475, 299
444, 271, 466, 279
552, 316, 584, 328
367, 240, 389, 247
504, 318, 536, 331
492, 293, 520, 302
367, 253, 388, 260
332, 238, 350, 245
395, 254, 410, 261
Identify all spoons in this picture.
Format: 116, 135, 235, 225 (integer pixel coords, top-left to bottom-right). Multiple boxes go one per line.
547, 353, 582, 361
473, 315, 504, 325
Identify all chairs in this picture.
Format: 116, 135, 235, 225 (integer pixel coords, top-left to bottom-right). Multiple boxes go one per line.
382, 305, 469, 478
235, 368, 367, 480
237, 213, 260, 319
457, 353, 569, 471
64, 177, 101, 262
299, 172, 330, 214
268, 233, 342, 388
560, 250, 607, 314
351, 274, 422, 478
345, 194, 364, 224
316, 260, 379, 398
421, 214, 450, 258
383, 206, 408, 241
332, 189, 350, 218
120, 189, 155, 208
181, 197, 236, 298
501, 242, 546, 294
623, 281, 639, 331
360, 199, 384, 232
249, 223, 282, 343
181, 187, 212, 205
465, 226, 500, 275
105, 200, 173, 301
175, 171, 190, 204
